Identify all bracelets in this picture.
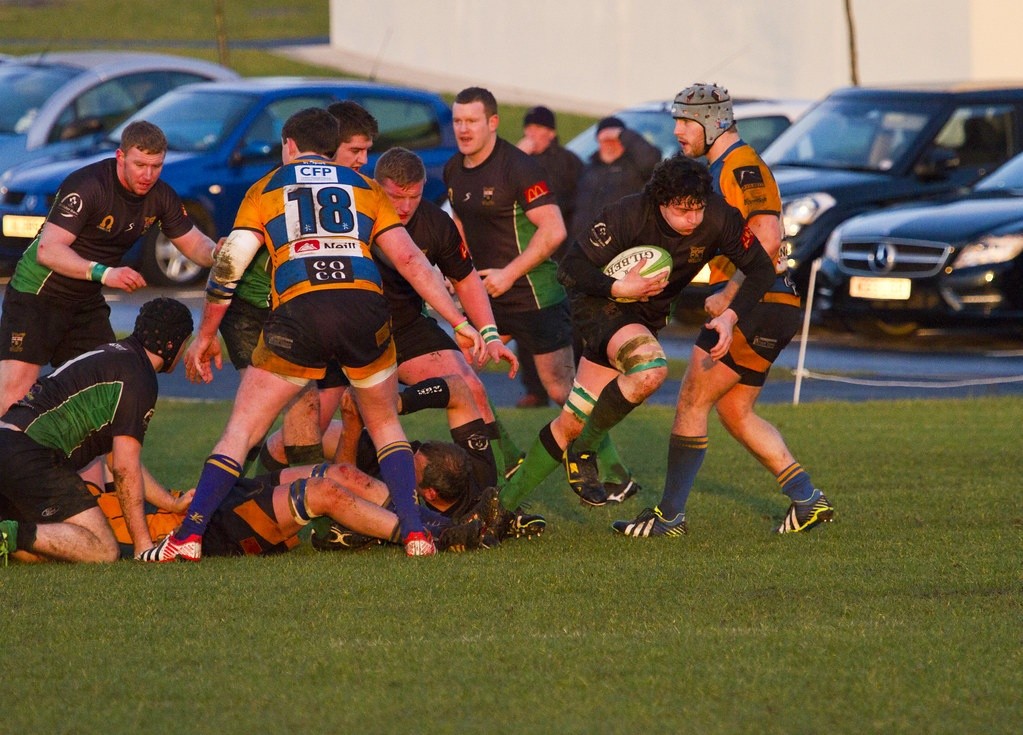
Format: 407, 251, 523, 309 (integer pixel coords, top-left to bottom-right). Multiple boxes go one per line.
452, 317, 467, 328
91, 262, 108, 284
479, 325, 502, 345
454, 321, 469, 333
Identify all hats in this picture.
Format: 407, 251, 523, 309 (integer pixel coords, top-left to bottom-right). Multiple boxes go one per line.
524, 106, 555, 129
671, 82, 734, 146
597, 117, 625, 132
132, 296, 195, 373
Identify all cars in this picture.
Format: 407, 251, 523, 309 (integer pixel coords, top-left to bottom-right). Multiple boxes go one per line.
0, 50, 287, 203
0, 75, 461, 290
562, 97, 896, 186
813, 151, 1022, 344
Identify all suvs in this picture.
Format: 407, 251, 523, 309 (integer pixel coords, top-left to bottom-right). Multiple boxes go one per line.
747, 80, 1023, 330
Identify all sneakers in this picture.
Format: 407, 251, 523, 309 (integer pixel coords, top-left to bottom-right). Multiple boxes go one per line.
611, 505, 690, 540
310, 522, 379, 554
138, 527, 202, 564
561, 438, 610, 506
0, 525, 11, 568
579, 472, 642, 508
503, 501, 547, 540
437, 517, 485, 555
403, 525, 438, 557
456, 482, 502, 546
775, 488, 836, 536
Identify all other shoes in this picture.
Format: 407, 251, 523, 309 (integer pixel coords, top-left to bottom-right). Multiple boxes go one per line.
515, 392, 549, 408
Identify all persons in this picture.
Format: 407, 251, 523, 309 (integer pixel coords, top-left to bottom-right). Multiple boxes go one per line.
477, 152, 776, 546
0, 86, 664, 565
611, 84, 834, 540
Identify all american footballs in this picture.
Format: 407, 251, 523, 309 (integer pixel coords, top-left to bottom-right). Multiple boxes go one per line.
603, 245, 673, 302
